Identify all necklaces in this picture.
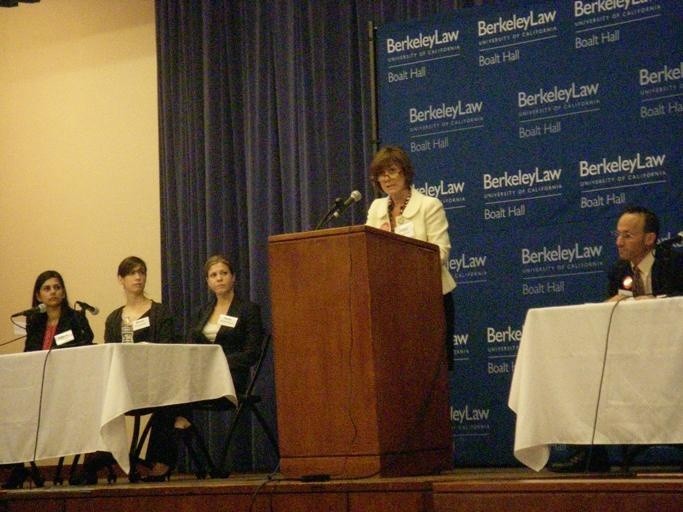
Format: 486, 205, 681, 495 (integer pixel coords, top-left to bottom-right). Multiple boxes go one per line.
389, 186, 411, 222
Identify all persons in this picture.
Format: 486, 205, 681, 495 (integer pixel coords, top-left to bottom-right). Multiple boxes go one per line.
68, 256, 174, 486
364, 146, 457, 371
141, 255, 263, 484
545, 208, 683, 473
0, 270, 94, 489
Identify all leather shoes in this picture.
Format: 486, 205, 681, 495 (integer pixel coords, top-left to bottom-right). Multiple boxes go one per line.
0, 465, 29, 491
548, 446, 607, 474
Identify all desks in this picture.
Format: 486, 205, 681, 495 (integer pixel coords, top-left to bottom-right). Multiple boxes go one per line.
0, 343, 238, 485
507, 295, 683, 473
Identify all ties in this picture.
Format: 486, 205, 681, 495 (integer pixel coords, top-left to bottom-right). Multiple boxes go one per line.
631, 266, 649, 298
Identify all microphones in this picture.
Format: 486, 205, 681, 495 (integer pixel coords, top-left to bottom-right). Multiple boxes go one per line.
329, 189, 362, 221
12, 303, 47, 317
77, 301, 99, 315
618, 276, 633, 297
655, 230, 683, 250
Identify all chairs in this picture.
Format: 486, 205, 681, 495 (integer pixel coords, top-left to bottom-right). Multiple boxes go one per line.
128, 331, 280, 479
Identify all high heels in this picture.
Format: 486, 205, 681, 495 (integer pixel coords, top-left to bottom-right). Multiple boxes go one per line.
161, 412, 198, 445
142, 457, 175, 484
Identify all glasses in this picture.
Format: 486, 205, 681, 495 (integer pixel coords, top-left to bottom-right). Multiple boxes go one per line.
373, 169, 404, 184
612, 230, 645, 240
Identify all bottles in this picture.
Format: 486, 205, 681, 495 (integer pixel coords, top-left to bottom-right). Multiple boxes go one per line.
120, 315, 134, 344
